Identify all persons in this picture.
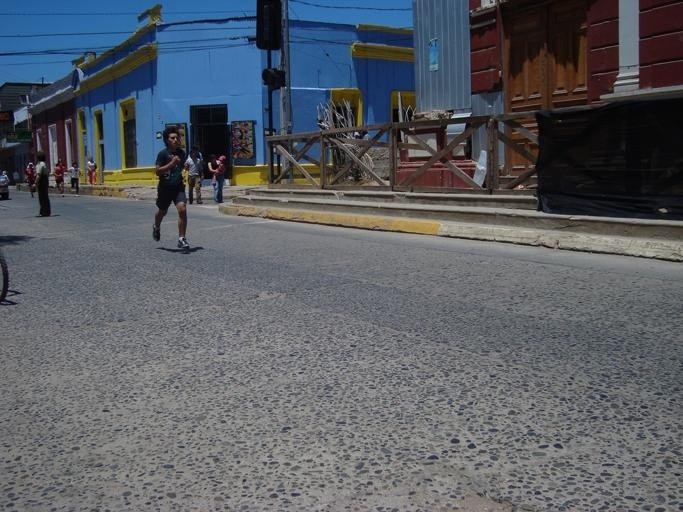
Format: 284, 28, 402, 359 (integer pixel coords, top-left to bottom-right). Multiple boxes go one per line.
208, 153, 227, 203
151, 126, 191, 248
53, 160, 67, 197
34, 149, 52, 217
211, 156, 226, 185
2, 171, 9, 185
187, 143, 206, 165
86, 156, 97, 185
66, 160, 83, 196
25, 163, 36, 197
184, 150, 204, 205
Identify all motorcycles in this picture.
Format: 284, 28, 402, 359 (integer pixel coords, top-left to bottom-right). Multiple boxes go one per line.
0, 175, 9, 200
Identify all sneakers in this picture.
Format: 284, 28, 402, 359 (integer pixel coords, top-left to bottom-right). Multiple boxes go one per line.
177, 238, 189, 248
152, 224, 160, 241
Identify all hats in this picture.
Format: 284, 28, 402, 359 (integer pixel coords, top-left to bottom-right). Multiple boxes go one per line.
219, 156, 225, 161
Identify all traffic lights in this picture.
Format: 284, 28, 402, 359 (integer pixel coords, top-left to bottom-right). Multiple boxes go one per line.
261, 66, 280, 90
